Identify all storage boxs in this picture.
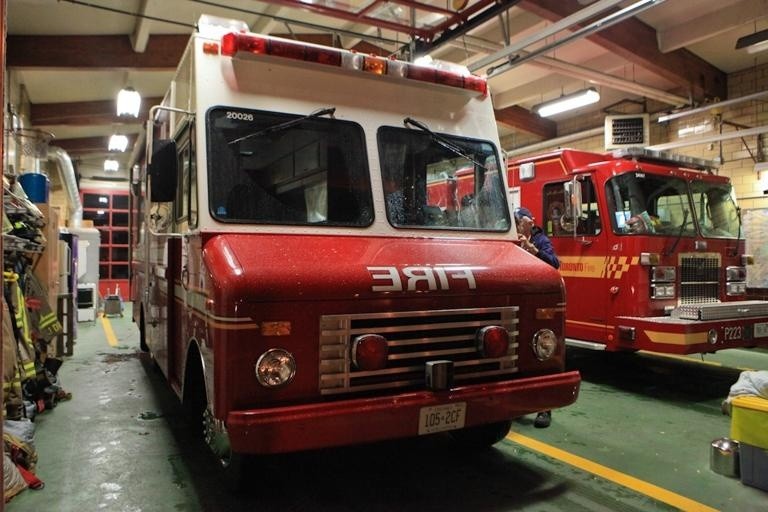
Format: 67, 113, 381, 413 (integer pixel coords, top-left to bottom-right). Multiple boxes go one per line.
730, 392, 768, 495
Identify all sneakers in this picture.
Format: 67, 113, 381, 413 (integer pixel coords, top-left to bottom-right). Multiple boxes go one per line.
534, 409, 551, 429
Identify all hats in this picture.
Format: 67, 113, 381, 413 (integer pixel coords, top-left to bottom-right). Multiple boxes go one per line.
514, 207, 532, 219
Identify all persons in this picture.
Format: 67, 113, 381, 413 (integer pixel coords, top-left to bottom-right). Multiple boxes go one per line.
511, 207, 562, 429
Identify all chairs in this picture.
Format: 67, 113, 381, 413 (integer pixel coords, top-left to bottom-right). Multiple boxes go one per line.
226, 179, 278, 220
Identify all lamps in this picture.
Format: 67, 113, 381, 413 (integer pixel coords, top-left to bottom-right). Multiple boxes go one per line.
535, 82, 600, 119
104, 86, 141, 171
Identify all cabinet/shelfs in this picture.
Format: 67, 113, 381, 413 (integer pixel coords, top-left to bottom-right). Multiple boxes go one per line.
3, 176, 100, 360
